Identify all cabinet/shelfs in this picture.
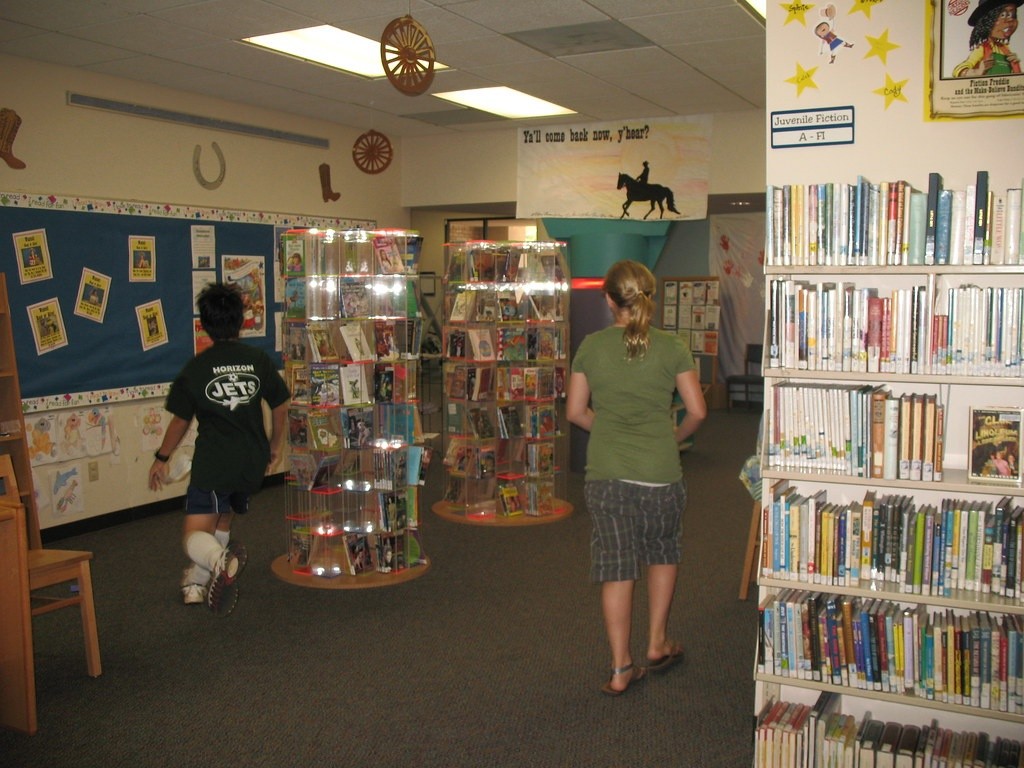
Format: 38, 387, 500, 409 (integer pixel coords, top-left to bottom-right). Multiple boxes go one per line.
751, 265, 1024, 768
0, 502, 37, 737
271, 224, 431, 590
0, 272, 43, 551
433, 240, 575, 527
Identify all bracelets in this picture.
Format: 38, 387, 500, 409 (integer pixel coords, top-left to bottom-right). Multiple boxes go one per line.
155, 451, 169, 461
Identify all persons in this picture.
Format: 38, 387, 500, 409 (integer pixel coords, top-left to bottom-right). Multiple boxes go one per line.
565, 259, 707, 696
148, 282, 290, 617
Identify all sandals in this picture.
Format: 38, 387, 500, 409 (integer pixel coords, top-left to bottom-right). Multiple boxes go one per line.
601, 661, 645, 698
646, 637, 687, 673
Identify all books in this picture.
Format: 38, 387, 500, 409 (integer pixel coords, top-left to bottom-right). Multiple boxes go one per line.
755, 172, 1024, 768
438, 241, 568, 515
275, 229, 429, 577
662, 281, 721, 384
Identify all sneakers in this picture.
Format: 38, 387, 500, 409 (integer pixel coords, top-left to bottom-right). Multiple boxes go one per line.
180, 568, 211, 605
207, 542, 248, 617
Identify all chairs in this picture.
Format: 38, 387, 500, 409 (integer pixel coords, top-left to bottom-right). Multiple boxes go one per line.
727, 344, 763, 410
0, 455, 102, 676
